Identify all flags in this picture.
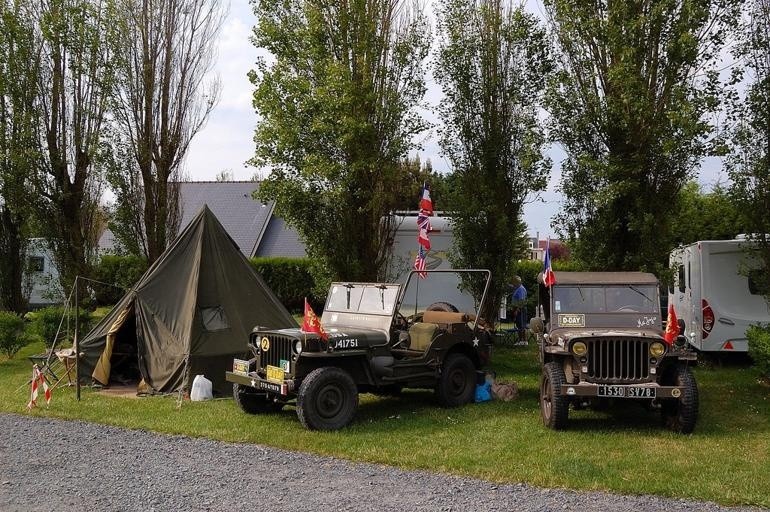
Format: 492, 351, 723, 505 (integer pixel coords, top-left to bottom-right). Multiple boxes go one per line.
27, 365, 52, 409
302, 303, 328, 341
414, 189, 434, 279
665, 304, 680, 346
543, 239, 556, 288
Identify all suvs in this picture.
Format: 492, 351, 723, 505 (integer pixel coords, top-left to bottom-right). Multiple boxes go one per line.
529, 270, 698, 431
223, 270, 493, 430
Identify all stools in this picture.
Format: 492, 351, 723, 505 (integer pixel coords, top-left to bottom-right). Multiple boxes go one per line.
499, 327, 536, 345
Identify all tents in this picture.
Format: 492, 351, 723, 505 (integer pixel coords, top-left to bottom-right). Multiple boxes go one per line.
58, 205, 302, 399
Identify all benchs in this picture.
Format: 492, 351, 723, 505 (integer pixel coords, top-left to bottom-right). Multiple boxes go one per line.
421, 310, 464, 331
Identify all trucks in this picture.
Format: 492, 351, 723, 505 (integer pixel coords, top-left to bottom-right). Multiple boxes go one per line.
668, 234, 770, 352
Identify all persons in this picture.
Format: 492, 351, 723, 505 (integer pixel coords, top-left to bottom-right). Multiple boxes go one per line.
511, 276, 529, 345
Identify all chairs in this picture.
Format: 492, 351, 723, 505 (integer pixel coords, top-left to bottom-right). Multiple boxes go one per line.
389, 321, 440, 358
28, 329, 67, 387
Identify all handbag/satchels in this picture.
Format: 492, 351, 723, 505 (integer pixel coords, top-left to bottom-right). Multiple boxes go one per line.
475, 381, 492, 402
492, 380, 520, 402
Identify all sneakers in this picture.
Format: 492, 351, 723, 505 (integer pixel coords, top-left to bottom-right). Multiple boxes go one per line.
514, 341, 529, 346
